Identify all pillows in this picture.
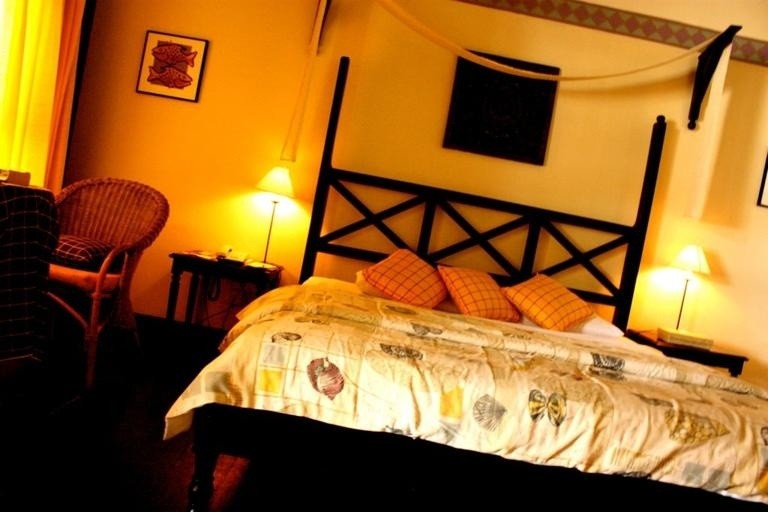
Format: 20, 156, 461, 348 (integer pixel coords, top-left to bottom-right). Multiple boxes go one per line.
355, 247, 628, 340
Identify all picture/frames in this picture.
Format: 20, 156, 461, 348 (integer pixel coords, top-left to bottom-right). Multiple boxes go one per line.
135, 29, 209, 102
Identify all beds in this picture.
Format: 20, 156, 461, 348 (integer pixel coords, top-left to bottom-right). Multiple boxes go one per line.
187, 58, 768, 512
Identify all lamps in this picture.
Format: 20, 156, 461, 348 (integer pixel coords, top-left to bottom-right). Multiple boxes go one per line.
674, 246, 710, 331
254, 165, 295, 264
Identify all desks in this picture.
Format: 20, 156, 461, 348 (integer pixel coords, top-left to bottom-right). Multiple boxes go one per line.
628, 326, 749, 380
163, 248, 284, 348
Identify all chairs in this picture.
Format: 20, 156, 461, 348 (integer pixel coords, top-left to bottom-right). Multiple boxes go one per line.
0, 177, 170, 386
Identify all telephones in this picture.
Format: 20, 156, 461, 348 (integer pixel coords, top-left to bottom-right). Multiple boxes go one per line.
217, 247, 247, 264
